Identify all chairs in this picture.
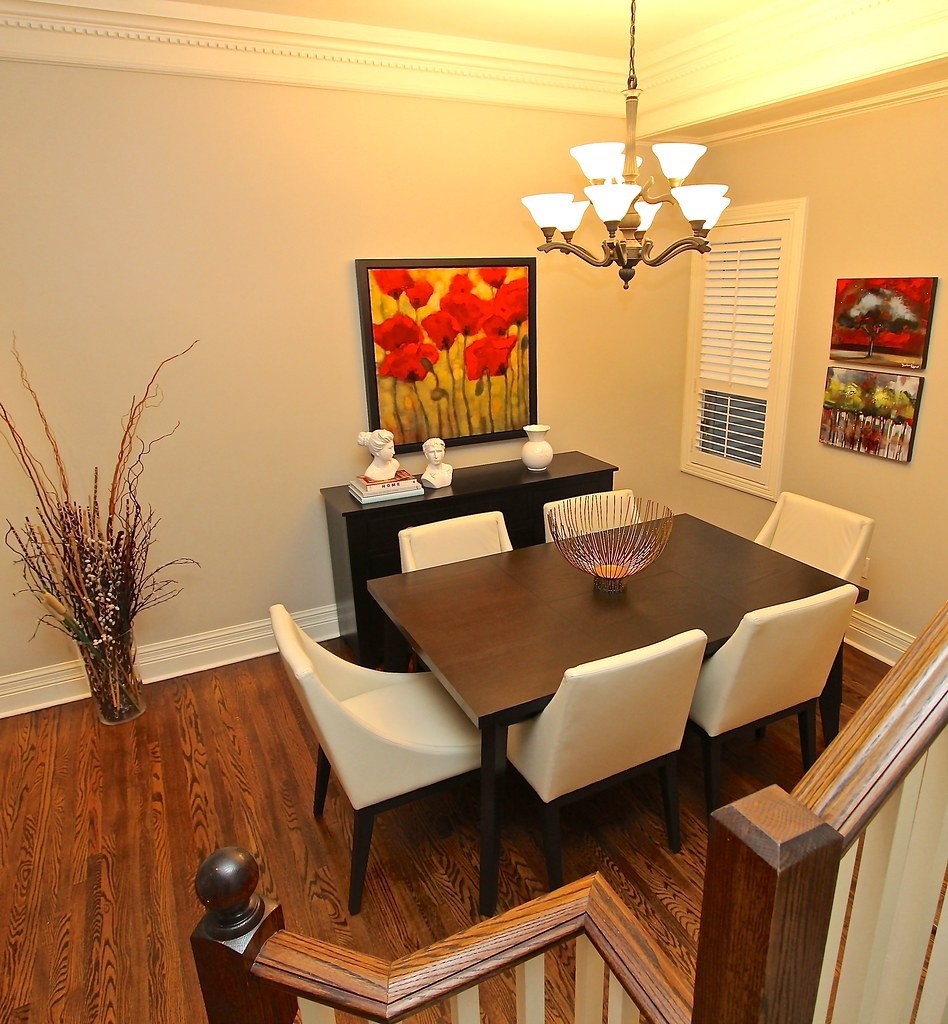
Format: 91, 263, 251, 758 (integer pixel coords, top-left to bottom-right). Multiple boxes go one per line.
689, 583, 859, 827
543, 488, 642, 546
753, 490, 876, 586
398, 510, 514, 574
271, 602, 508, 915
503, 628, 709, 893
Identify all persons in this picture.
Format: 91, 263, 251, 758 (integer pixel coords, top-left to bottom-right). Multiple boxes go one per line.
420, 438, 452, 489
357, 429, 400, 481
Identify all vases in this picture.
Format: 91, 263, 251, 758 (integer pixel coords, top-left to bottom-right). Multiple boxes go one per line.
521, 424, 553, 471
75, 620, 147, 726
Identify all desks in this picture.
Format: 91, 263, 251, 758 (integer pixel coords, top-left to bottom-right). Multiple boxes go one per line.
318, 451, 619, 665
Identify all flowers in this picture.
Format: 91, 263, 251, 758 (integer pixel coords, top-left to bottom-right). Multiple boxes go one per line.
1, 328, 202, 718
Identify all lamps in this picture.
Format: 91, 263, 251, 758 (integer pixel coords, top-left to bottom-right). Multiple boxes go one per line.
520, 0, 732, 291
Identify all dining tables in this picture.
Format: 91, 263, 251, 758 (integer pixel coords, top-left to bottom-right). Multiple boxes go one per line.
366, 512, 871, 918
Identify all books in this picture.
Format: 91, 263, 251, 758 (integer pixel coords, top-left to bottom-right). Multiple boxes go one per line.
349, 469, 424, 504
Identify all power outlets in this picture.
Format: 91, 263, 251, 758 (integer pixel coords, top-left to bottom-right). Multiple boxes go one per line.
862, 556, 870, 580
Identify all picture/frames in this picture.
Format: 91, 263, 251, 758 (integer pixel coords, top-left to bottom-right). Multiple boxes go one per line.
819, 366, 926, 463
829, 275, 938, 371
354, 256, 538, 458
